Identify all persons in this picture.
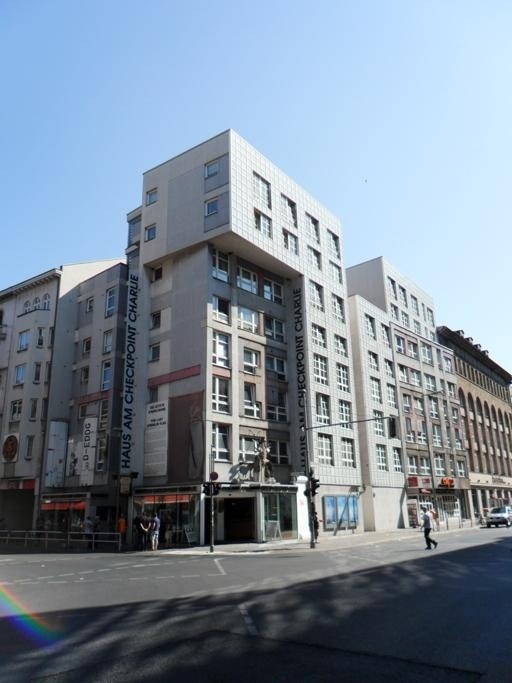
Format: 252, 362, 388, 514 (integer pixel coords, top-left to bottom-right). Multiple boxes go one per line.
83, 516, 94, 549
132, 511, 161, 552
92, 516, 101, 549
165, 514, 174, 548
419, 507, 438, 552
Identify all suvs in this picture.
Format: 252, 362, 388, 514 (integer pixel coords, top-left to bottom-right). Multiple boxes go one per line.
486, 506, 512, 527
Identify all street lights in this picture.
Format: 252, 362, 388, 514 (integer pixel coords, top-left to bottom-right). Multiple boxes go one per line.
302, 389, 391, 549
